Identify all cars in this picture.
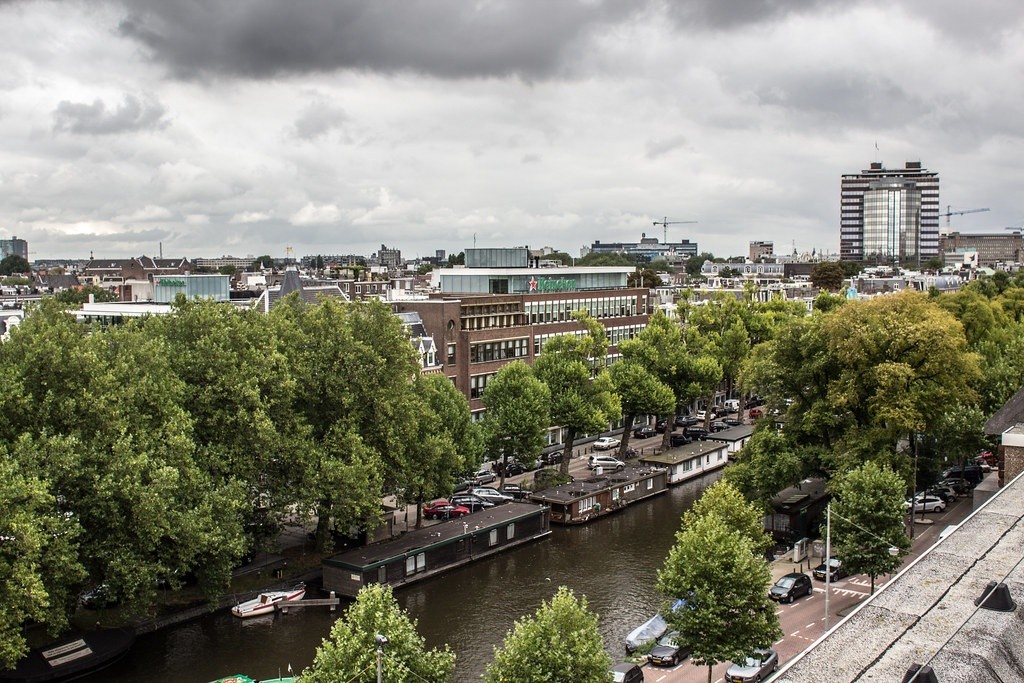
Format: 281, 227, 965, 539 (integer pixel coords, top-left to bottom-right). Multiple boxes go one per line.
768, 573, 813, 604
402, 397, 794, 519
902, 494, 946, 515
916, 447, 998, 505
81, 584, 119, 609
606, 662, 645, 683
647, 629, 696, 667
724, 648, 779, 683
812, 555, 857, 583
242, 512, 281, 534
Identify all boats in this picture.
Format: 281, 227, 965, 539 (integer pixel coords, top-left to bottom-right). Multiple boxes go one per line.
626, 589, 703, 654
319, 421, 785, 600
230, 581, 307, 618
762, 475, 836, 561
207, 667, 303, 683
0, 625, 136, 683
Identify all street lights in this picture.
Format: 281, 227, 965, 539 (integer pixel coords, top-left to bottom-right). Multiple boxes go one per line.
372, 633, 388, 683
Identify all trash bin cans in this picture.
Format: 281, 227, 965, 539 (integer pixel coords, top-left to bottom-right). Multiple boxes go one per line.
274, 567, 283, 578
794, 537, 809, 563
812, 540, 826, 557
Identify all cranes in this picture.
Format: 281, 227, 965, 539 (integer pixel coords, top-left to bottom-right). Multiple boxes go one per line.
654, 216, 698, 245
940, 204, 990, 234
